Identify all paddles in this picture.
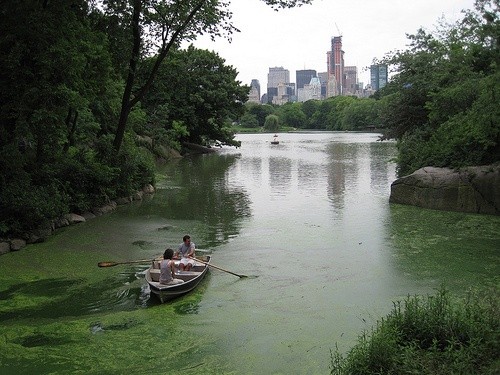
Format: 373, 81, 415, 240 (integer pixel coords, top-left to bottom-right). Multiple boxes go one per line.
184, 254, 248, 279
97, 257, 182, 268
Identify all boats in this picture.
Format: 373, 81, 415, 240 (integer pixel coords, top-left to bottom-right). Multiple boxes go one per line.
145, 252, 211, 304
271, 139, 280, 145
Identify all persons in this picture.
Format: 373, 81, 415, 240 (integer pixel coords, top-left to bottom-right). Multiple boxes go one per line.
159, 248, 184, 285
175, 235, 195, 271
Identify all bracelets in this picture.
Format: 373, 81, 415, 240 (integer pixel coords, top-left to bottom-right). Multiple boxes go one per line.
188, 254, 190, 256
